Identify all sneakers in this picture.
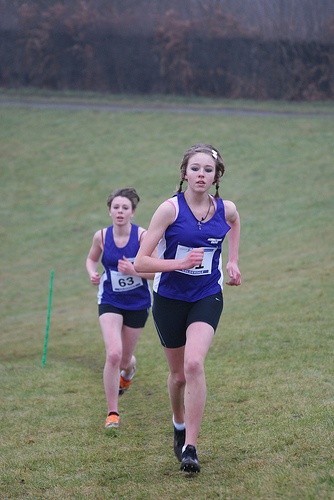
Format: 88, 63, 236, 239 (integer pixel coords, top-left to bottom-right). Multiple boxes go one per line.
179, 444, 201, 474
173, 425, 186, 462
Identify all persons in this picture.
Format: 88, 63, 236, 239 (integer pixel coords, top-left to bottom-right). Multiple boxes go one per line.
133, 143, 241, 473
87, 187, 159, 429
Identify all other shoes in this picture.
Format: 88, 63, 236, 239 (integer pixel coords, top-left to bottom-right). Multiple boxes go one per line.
104, 411, 120, 430
118, 364, 136, 397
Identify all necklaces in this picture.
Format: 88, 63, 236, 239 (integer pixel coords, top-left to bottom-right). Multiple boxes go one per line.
197, 195, 212, 230
